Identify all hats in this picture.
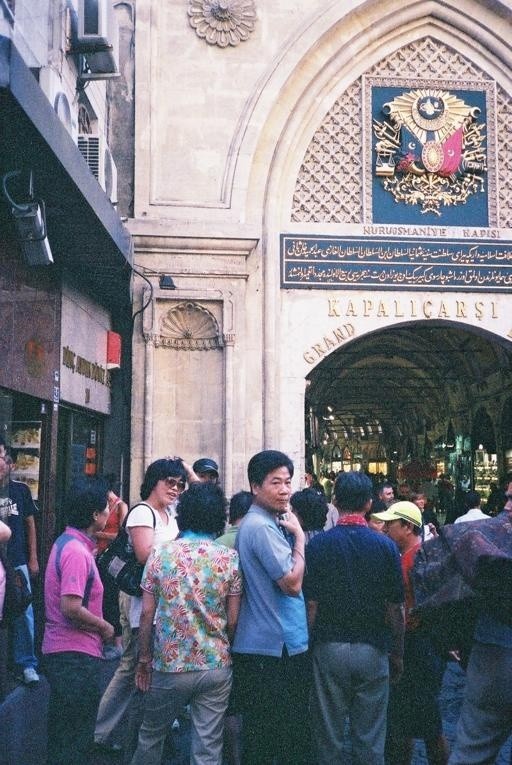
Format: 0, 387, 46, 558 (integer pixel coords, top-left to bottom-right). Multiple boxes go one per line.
370, 500, 423, 528
193, 457, 219, 477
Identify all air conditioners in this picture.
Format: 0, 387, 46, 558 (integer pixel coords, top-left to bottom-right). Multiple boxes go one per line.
77, 0, 120, 73
78, 133, 118, 213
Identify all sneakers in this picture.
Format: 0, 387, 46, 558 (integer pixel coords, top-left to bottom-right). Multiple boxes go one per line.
102, 642, 124, 661
21, 667, 39, 685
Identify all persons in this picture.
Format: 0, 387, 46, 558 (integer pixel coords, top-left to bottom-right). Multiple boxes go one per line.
0, 440, 512, 765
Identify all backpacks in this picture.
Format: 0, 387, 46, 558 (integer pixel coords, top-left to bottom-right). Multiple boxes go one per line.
3, 568, 33, 625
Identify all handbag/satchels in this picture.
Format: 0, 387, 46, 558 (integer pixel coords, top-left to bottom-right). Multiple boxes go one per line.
94, 535, 145, 598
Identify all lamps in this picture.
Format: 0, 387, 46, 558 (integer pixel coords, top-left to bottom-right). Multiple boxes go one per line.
133, 263, 178, 290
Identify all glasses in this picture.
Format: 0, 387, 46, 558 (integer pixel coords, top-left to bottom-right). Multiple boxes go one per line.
163, 478, 186, 492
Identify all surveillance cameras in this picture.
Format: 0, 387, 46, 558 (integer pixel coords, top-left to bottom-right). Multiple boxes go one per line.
10, 191, 55, 267
